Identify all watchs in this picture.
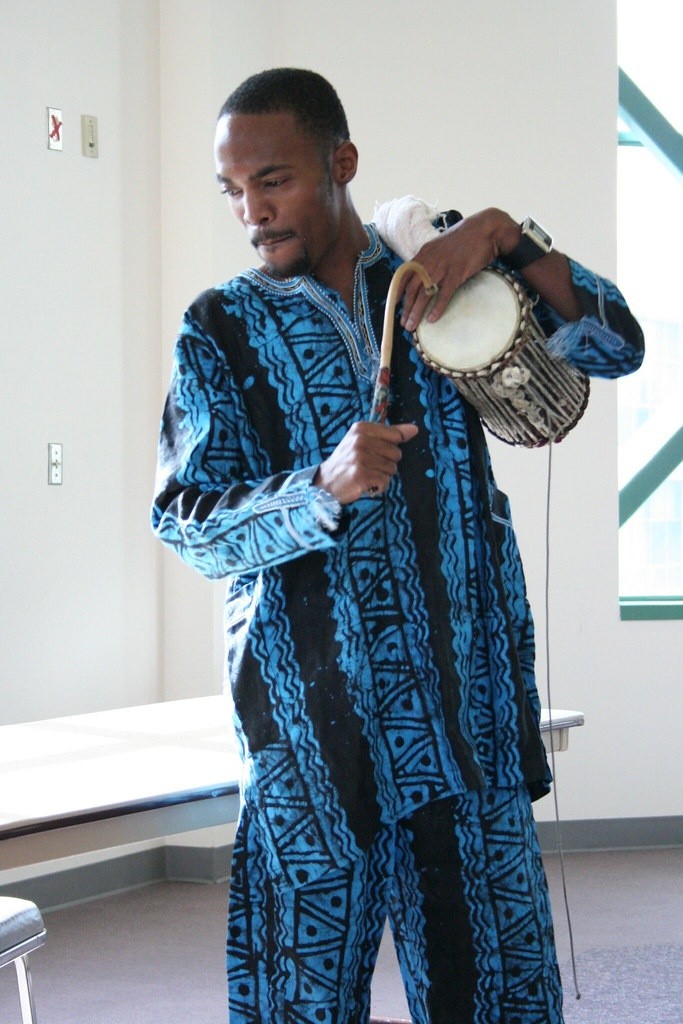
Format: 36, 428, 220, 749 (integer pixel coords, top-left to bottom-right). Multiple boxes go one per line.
501, 214, 553, 271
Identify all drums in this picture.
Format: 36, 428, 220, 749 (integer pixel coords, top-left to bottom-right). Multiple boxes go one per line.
411, 259, 591, 448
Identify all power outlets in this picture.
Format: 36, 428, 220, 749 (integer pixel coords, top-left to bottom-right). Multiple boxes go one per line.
47, 442, 63, 486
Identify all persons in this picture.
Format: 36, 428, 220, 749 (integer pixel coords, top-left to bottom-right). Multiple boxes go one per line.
152, 68, 644, 1024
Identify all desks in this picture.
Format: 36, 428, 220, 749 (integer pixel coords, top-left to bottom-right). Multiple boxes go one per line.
0, 695, 585, 842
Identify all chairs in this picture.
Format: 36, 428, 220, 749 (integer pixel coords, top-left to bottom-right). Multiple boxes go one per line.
0, 895, 49, 1024
